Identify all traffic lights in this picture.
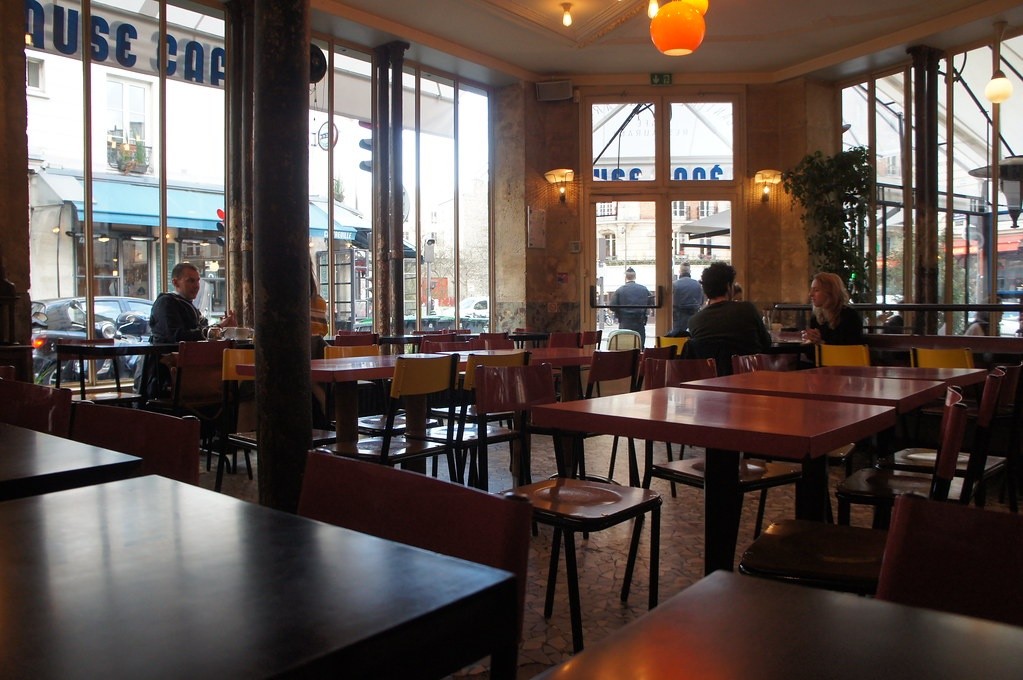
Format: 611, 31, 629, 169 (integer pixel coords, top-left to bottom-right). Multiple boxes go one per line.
423, 238, 437, 263
215, 206, 227, 248
357, 119, 374, 174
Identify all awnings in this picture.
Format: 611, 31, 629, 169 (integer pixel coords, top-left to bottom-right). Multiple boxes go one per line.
72, 181, 358, 240
680, 208, 731, 240
953, 233, 1023, 256
352, 228, 423, 258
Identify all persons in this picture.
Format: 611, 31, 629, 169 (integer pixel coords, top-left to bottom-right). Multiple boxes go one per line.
609, 267, 656, 350
881, 300, 918, 368
149, 263, 240, 454
310, 255, 328, 423
854, 293, 893, 334
733, 282, 743, 302
672, 261, 705, 330
960, 296, 1004, 370
689, 259, 772, 377
803, 273, 863, 362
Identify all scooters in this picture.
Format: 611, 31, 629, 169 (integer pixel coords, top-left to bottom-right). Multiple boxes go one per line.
604, 309, 613, 326
69, 300, 149, 377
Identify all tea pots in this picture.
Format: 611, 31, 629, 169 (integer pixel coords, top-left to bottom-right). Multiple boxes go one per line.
201, 327, 228, 342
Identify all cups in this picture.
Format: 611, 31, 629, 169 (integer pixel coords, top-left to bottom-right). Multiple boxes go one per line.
236, 328, 254, 340
223, 327, 237, 339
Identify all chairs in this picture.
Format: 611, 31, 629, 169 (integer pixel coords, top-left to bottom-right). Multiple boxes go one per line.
147, 328, 1023, 652
1, 378, 71, 439
71, 401, 202, 488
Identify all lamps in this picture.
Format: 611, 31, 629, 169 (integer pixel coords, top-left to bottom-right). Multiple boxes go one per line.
651, 0, 708, 55
755, 169, 785, 201
648, 0, 659, 18
544, 168, 578, 204
561, 3, 573, 27
986, 21, 1011, 103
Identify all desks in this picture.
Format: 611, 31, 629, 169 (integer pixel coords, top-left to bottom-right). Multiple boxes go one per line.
0, 347, 1022, 680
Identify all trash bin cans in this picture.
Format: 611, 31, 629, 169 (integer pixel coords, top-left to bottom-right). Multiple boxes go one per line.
354, 315, 489, 355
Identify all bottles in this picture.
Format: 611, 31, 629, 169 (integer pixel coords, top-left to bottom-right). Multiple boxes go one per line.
762, 310, 771, 331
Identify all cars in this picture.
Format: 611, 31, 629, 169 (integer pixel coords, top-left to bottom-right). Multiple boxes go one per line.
32, 295, 158, 385
441, 296, 490, 321
876, 294, 945, 329
962, 300, 1020, 337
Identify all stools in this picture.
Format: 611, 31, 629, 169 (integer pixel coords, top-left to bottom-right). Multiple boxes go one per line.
55, 338, 120, 399
